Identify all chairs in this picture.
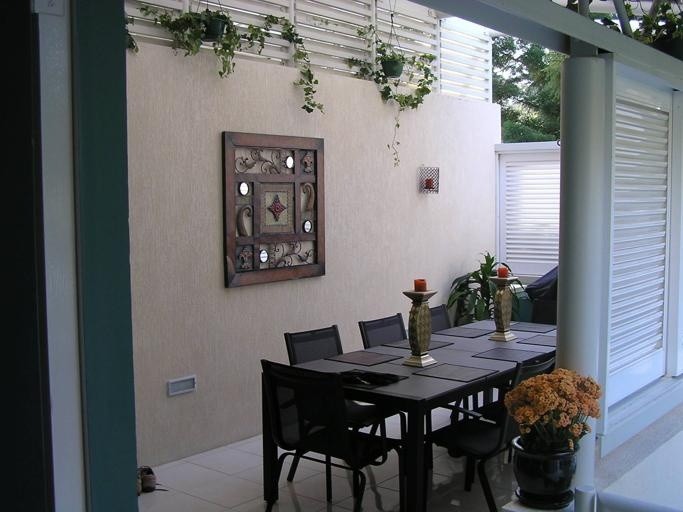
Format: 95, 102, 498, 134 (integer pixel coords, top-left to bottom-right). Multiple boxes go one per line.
359, 313, 458, 438
259, 359, 407, 512
431, 304, 503, 419
425, 355, 556, 510
283, 325, 409, 498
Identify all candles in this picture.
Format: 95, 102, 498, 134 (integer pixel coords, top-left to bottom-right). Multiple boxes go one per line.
496, 266, 508, 278
414, 278, 426, 292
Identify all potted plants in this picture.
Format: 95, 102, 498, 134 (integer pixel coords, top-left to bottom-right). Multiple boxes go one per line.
344, 24, 437, 168
503, 370, 603, 510
123, 5, 326, 113
